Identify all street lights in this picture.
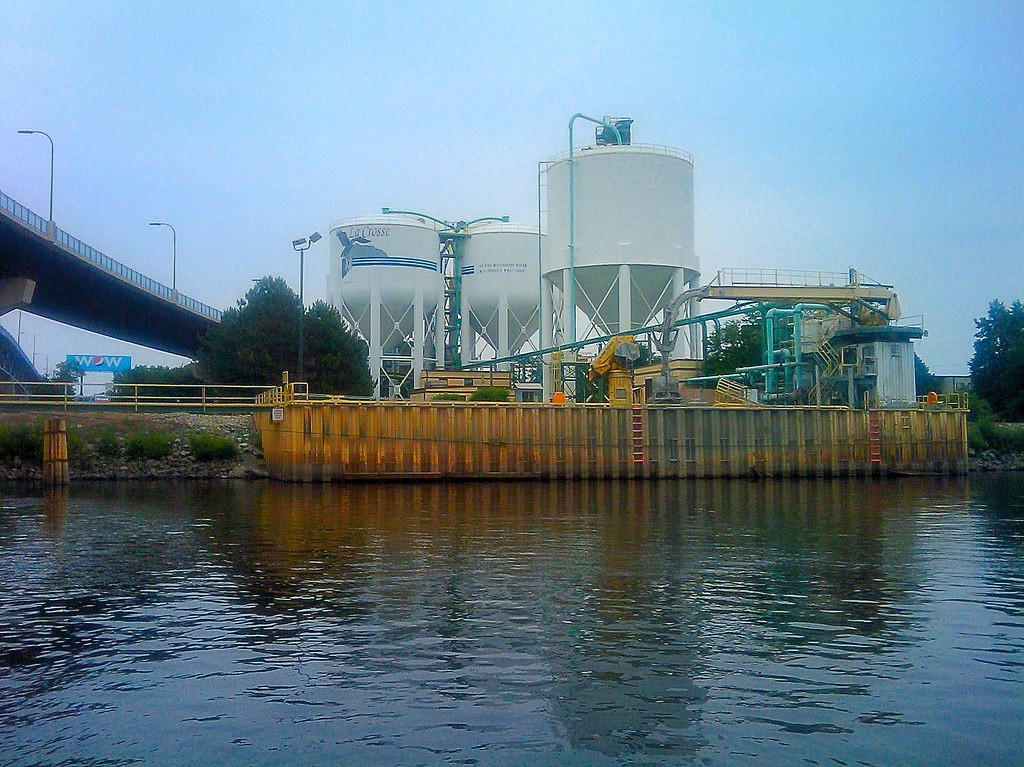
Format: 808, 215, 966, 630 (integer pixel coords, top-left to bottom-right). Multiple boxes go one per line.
293, 232, 323, 393
18, 130, 54, 220
150, 222, 175, 288
